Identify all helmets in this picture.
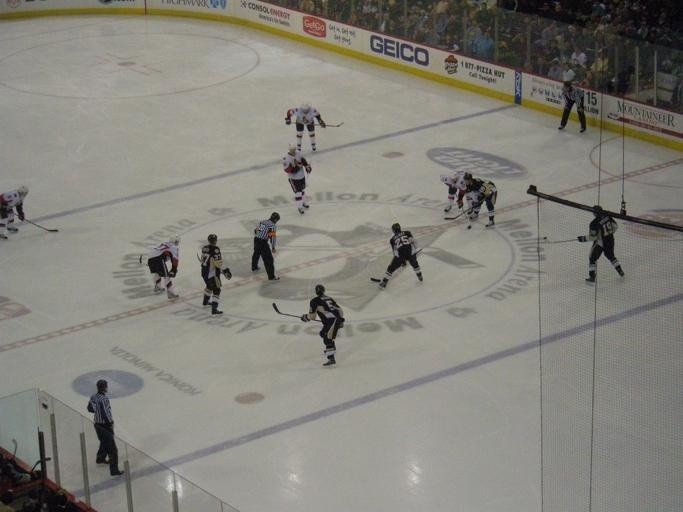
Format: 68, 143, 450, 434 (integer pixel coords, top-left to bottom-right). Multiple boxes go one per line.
207, 233, 217, 243
592, 205, 603, 214
314, 284, 326, 295
271, 212, 281, 222
463, 173, 473, 180
392, 223, 401, 235
17, 185, 29, 193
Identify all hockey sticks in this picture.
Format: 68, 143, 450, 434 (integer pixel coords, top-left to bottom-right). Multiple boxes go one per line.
291, 120, 344, 127
14, 211, 59, 232
455, 192, 472, 230
196, 250, 225, 275
544, 236, 579, 243
272, 302, 323, 323
371, 248, 422, 282
444, 198, 484, 220
138, 254, 150, 267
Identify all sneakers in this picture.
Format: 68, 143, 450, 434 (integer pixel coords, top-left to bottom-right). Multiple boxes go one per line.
202, 301, 213, 306
557, 123, 587, 133
211, 309, 224, 315
153, 286, 180, 300
95, 459, 110, 464
380, 283, 387, 288
322, 359, 336, 366
324, 347, 336, 353
110, 470, 125, 476
444, 200, 498, 227
298, 201, 309, 214
618, 270, 625, 276
0, 226, 19, 240
584, 275, 596, 282
416, 276, 424, 282
268, 275, 280, 280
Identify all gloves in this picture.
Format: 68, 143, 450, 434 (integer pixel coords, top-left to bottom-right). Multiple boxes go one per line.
222, 267, 232, 281
17, 212, 25, 221
577, 235, 587, 243
169, 267, 178, 278
318, 119, 326, 128
285, 117, 291, 125
305, 165, 312, 173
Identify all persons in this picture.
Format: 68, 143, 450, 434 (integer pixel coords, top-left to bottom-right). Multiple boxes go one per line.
285, 103, 327, 152
381, 223, 422, 287
198, 233, 232, 316
280, 143, 313, 214
579, 206, 625, 283
301, 283, 346, 365
0, 450, 82, 511
1, 185, 29, 241
87, 380, 125, 476
463, 172, 499, 227
250, 211, 281, 281
146, 233, 182, 300
437, 168, 470, 212
